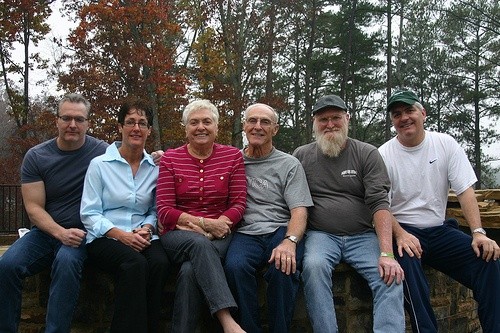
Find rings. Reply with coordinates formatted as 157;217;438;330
222;236;225;239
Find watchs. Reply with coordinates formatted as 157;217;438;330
281;236;301;246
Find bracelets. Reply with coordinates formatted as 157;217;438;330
473;228;486;235
379;251;394;259
143;225;153;241
204;232;215;241
199;216;208;231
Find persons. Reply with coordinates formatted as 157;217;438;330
224;102;315;333
375;90;500;333
80;103;170;333
0;93;163;333
292;95;408;333
158;99;248;333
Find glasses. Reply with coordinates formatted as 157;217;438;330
120;120;149;129
245;118;276;127
57;114;88;124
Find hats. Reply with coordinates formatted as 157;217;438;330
387;90;419;112
312;95;348;115
